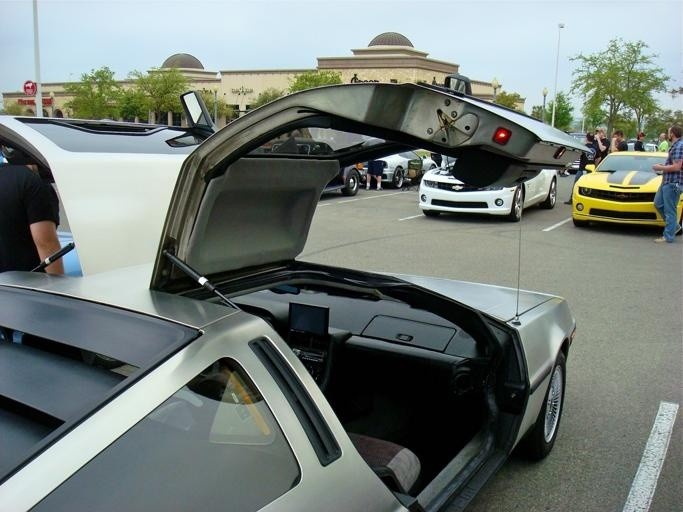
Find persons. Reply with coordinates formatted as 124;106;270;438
652;123;683;242
560;127;646;205
0;156;65;275
366;160;384;191
655;132;668;153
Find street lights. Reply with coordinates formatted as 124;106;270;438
551;22;567;127
490;78;499;104
541;86;549;123
212;83;218;127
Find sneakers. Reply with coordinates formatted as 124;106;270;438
674;224;682;236
653;235;667;244
563;199;572;205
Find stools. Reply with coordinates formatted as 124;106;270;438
344;427;426;498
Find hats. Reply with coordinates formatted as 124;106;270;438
637;132;646;137
585;132;595;142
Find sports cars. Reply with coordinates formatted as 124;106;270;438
0;74;595;512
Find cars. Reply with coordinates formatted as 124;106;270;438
416;159;560;222
624;142;659;152
270;137;361;197
357;151;438;189
571;150;683;235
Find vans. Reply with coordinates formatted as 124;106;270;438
566;132;588;172
